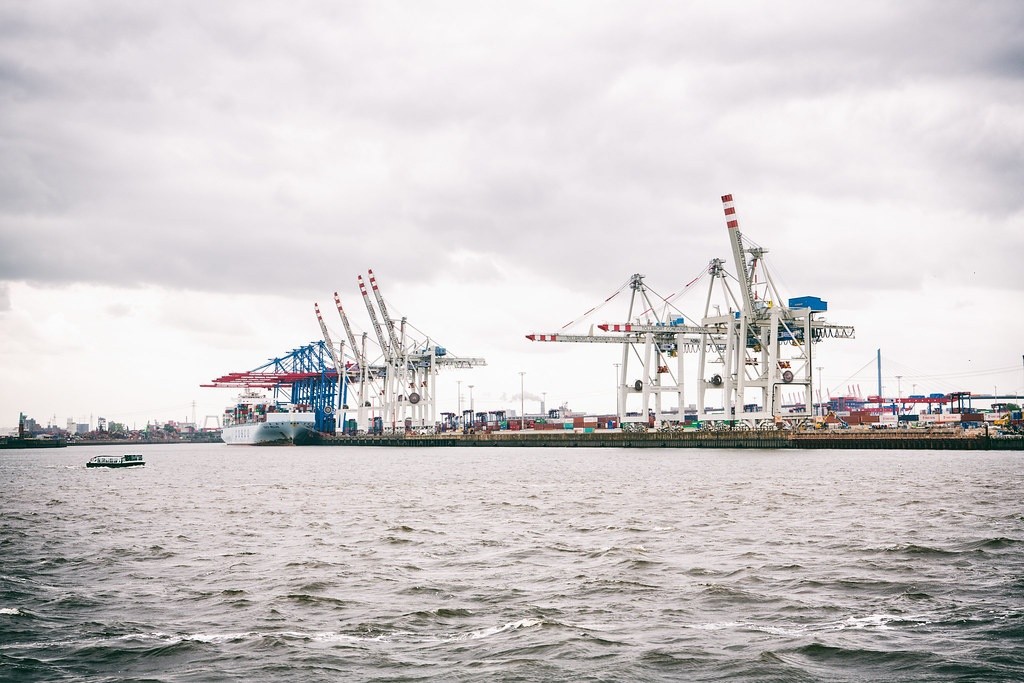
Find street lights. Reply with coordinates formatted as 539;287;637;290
456;379;462;418
896;376;903;398
613;362;621;413
518;369;527;419
816;366;825;403
468;384;476;412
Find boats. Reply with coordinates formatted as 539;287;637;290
0;423;68;448
220;392;317;446
85;453;147;470
